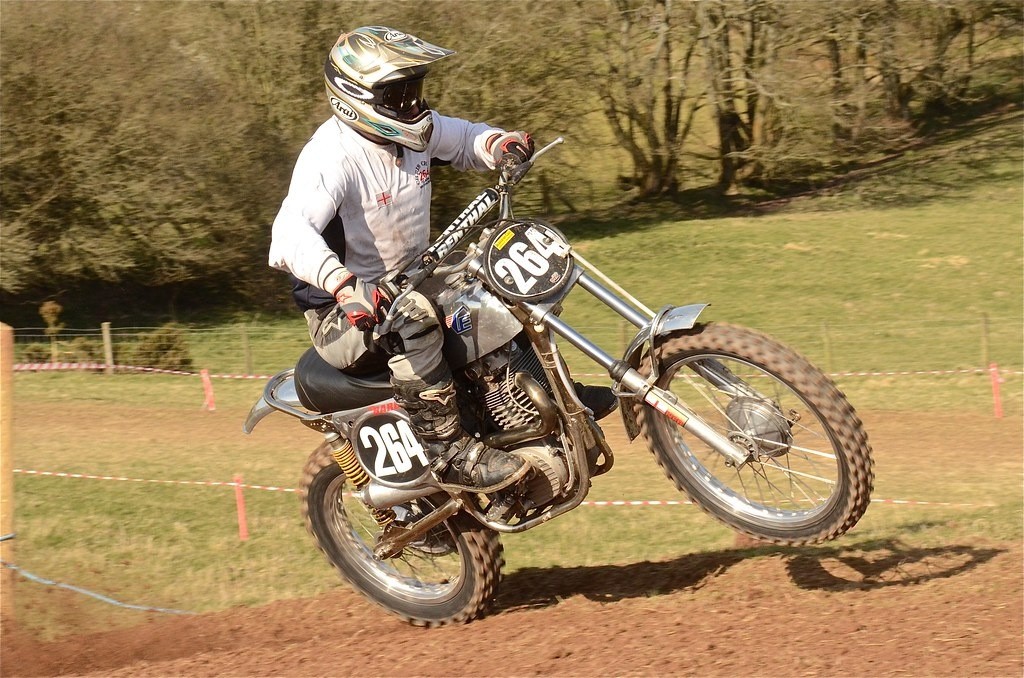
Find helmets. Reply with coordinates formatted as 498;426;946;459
323;24;456;153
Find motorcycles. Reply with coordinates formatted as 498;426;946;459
244;131;875;629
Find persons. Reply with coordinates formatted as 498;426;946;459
269;26;618;493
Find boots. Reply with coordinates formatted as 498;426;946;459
572;379;619;421
390;375;529;493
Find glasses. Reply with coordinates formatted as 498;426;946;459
383;77;421;113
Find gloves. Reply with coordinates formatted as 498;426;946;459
485;131;534;172
332;273;390;333
